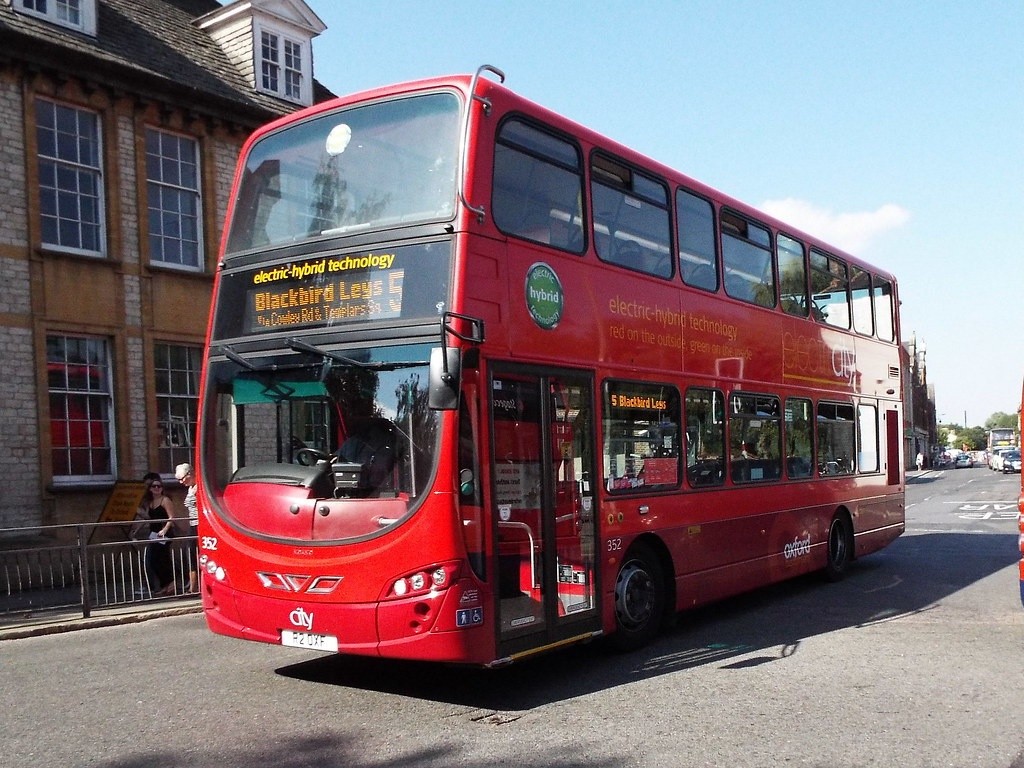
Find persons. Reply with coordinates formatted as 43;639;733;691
290;413;393;494
175;463;197;598
129;472;176;596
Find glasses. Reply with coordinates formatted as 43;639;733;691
178;473;188;481
151;484;162;489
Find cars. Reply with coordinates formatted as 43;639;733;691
1003;450;1022;474
988;446;1015;471
956;455;973;469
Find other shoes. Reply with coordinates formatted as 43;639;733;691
134;587;154;594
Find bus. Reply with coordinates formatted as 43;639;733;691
194;64;907;667
987;428;1016;469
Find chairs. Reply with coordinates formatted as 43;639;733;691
499;208;820;321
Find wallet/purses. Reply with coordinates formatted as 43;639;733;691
149;531;168;545
